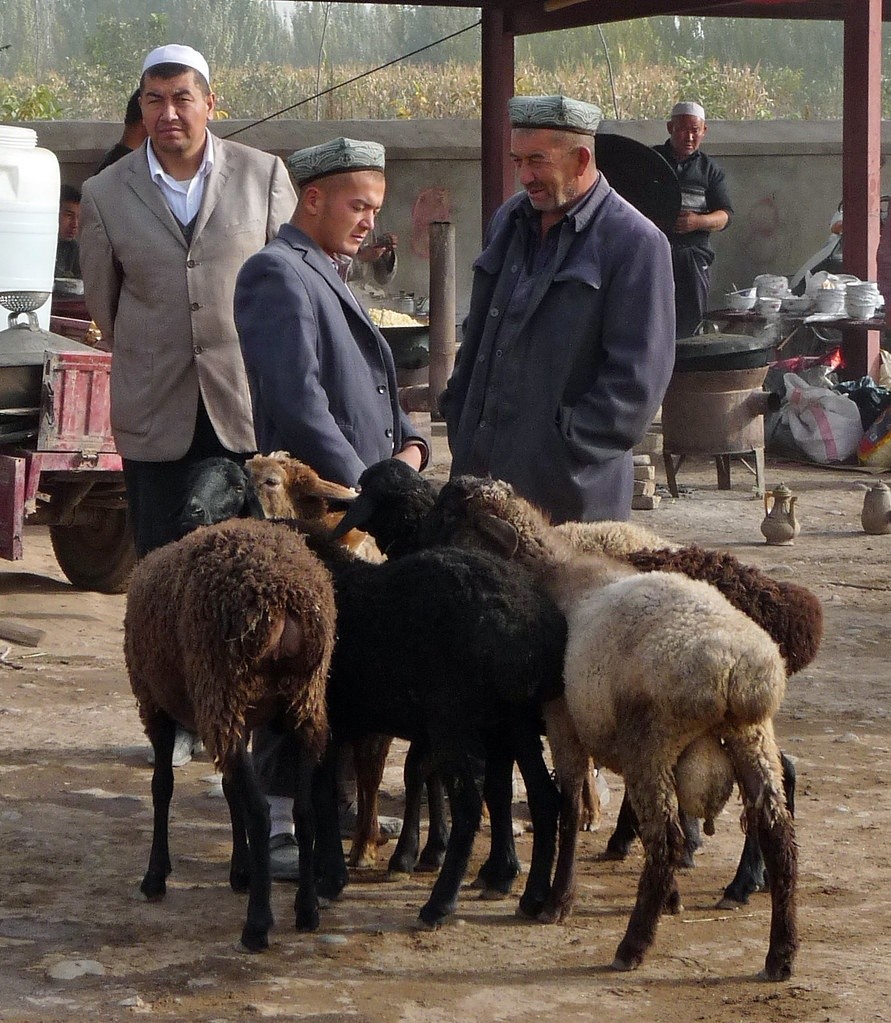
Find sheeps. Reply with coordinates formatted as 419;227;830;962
124;451;820;983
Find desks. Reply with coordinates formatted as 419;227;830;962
702;306;888;386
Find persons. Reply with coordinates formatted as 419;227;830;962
646;98;731;339
53;184;86;280
94;90;147;180
232;136;433;886
439;92;674;831
80;41;301;563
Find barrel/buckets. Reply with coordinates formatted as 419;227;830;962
0;122;63;337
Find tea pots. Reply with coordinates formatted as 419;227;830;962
760;482;800;546
862;479;891;535
387;290;428;317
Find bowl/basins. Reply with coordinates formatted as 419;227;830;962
723;293;758;312
752;271;879;321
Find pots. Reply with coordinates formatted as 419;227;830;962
672;319;777;373
359;292;387;315
590;130;683;240
1;310;107;410
374;322;432;370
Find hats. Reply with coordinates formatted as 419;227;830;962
141;44;209;85
671;102;705;119
286;135;386;188
508;94;601;136
